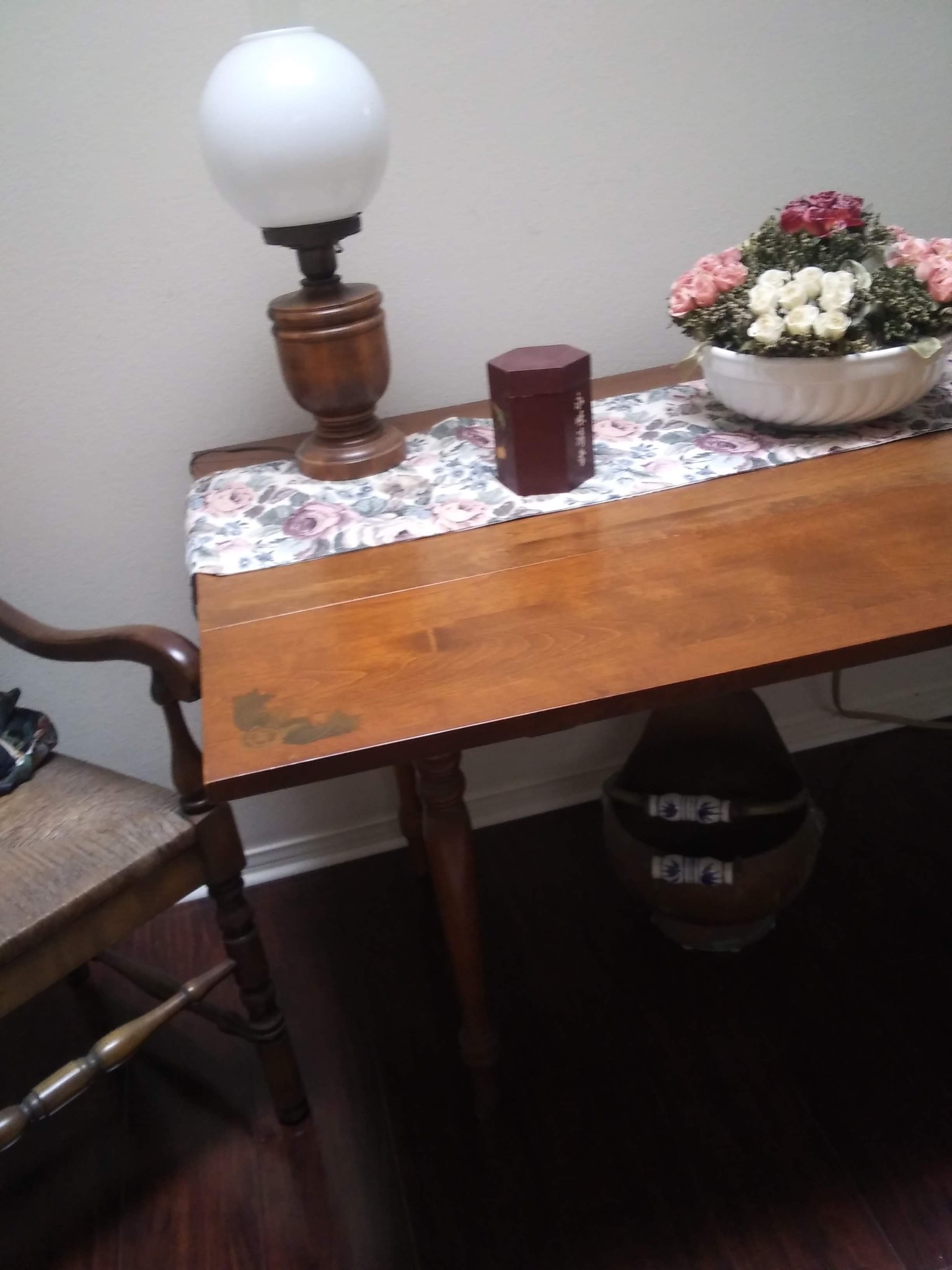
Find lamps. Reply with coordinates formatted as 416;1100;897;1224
202;26;407;478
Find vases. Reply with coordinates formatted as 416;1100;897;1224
699;332;948;427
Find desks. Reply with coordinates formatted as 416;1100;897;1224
187;359;952;1113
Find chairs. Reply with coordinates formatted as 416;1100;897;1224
0;601;307;1170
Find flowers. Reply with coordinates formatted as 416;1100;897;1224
671;187;952;357
